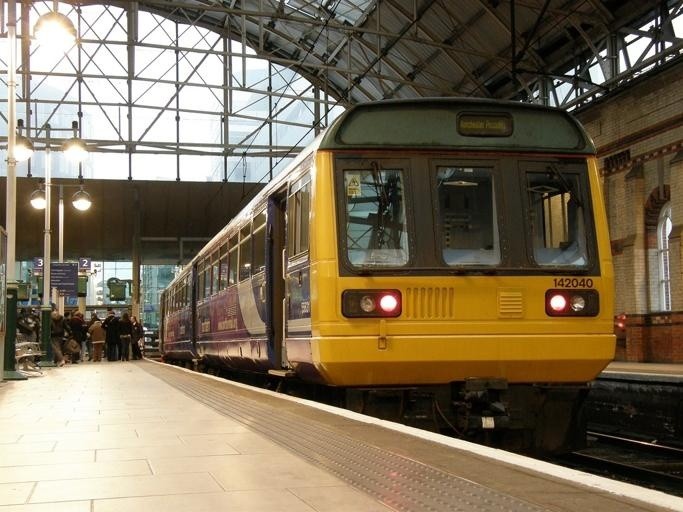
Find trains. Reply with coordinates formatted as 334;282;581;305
158;97;617;459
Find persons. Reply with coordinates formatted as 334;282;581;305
63;306;143;365
48;305;72;367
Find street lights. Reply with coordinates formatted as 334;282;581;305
3;0;77;380
30;177;92;263
16;118;89;367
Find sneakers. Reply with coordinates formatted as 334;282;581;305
53;354;143;368
